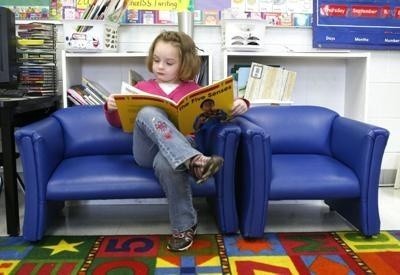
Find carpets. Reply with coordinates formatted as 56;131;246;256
0;229;400;275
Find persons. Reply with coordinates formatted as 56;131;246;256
103;28;252;252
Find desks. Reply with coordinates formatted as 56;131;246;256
0;94;68;236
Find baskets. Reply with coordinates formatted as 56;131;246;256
219;17;267;52
62;19;120;53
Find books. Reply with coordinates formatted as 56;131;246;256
112;75;235;136
66;0;130;48
67;69;145;107
230;60;298;104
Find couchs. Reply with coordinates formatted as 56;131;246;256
14;103;241;243
236;105;391;237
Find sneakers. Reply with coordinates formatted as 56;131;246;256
188;153;225;184
166;223;198;252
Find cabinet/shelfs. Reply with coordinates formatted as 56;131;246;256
222;48;372;123
61;49;213;110
